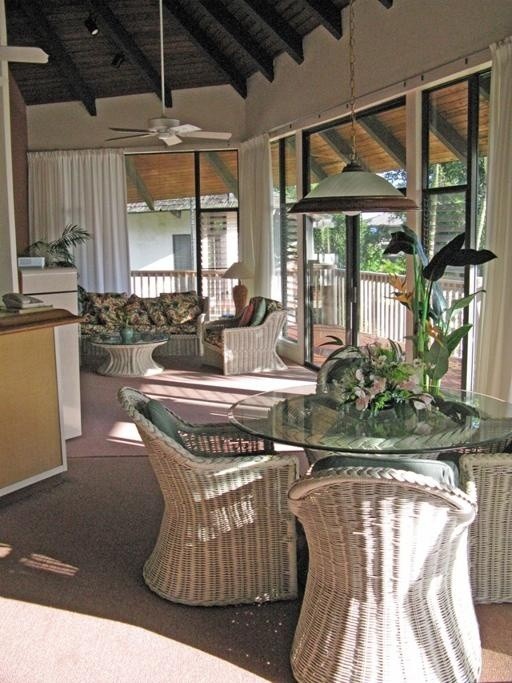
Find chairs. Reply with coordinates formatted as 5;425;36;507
202;298;288;374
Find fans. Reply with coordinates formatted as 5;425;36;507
106;2;231;145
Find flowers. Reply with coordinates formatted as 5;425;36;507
319;228;498;414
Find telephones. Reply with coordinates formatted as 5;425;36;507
2;293;53;309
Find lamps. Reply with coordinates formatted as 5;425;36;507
83;5;104;36
289;2;417;215
223;262;257;317
107;43;126;68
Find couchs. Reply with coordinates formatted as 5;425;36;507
78;293;211;370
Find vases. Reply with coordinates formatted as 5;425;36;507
119;317;134;342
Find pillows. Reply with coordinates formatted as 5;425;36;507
95;289;200;326
239;296;265;326
